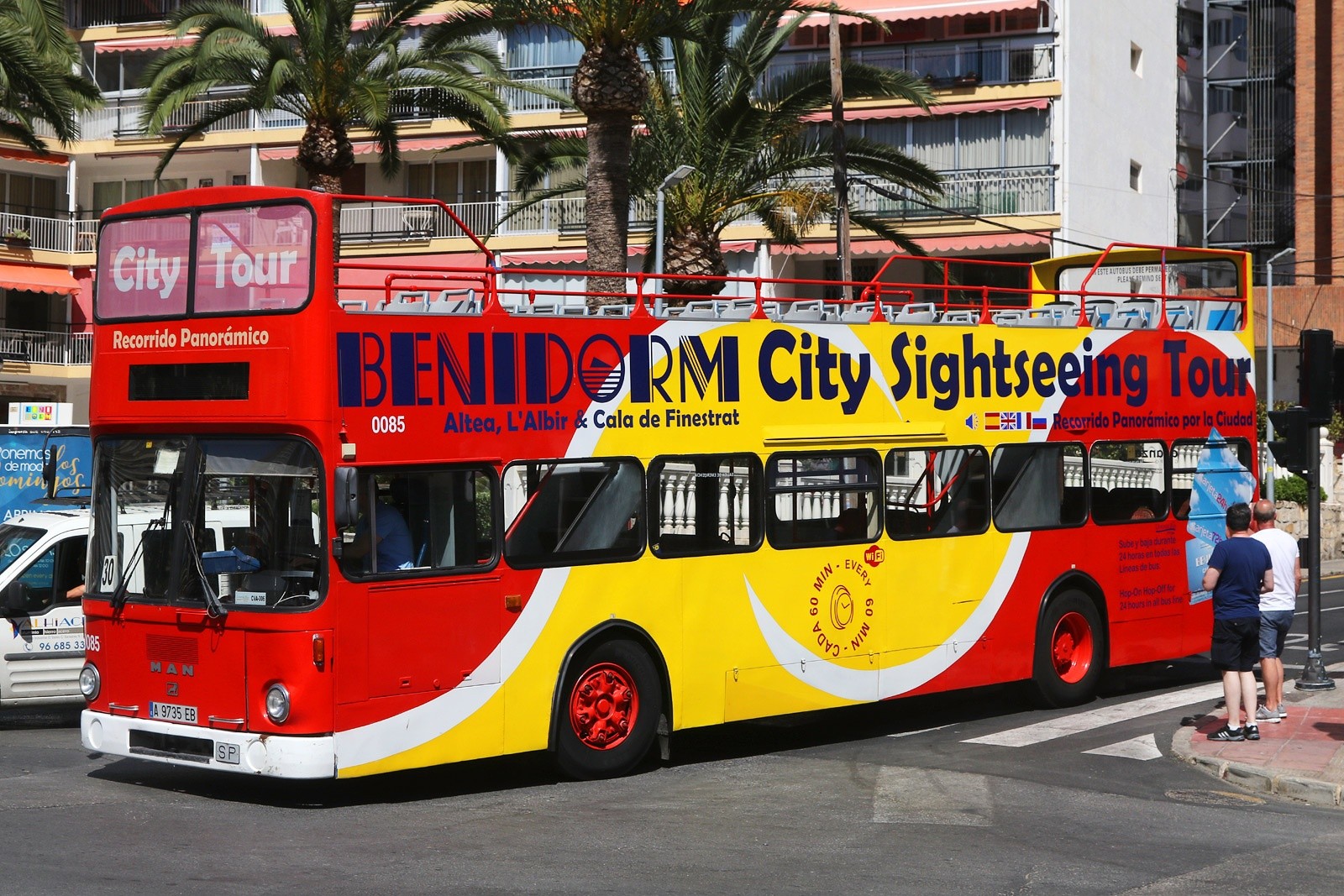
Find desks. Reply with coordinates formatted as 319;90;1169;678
77;231;97;253
0;332;45;360
401;210;440;242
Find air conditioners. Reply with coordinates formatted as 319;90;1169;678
1009;49;1050;79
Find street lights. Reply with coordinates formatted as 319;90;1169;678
654;166;696;318
1265;248;1296;503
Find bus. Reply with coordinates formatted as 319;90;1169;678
42;188;1260;777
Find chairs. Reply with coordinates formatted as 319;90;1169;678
1107;487;1161;521
257;279;1243;330
1061;485;1108;524
1161;489;1191;518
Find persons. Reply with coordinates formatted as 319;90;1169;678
1202;503;1274;741
339;478;415;576
1249;500;1302;723
818;509;865;540
54;547;85;603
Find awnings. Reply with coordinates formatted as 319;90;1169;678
0;262;82;295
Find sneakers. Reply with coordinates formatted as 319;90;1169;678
1245;704;1288;723
1207;725;1245;741
1243;725;1260;740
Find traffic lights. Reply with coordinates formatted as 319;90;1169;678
1267;407;1309;471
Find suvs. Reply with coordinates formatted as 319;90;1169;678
1;486;319;707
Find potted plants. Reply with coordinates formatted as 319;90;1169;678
0;227;32;248
